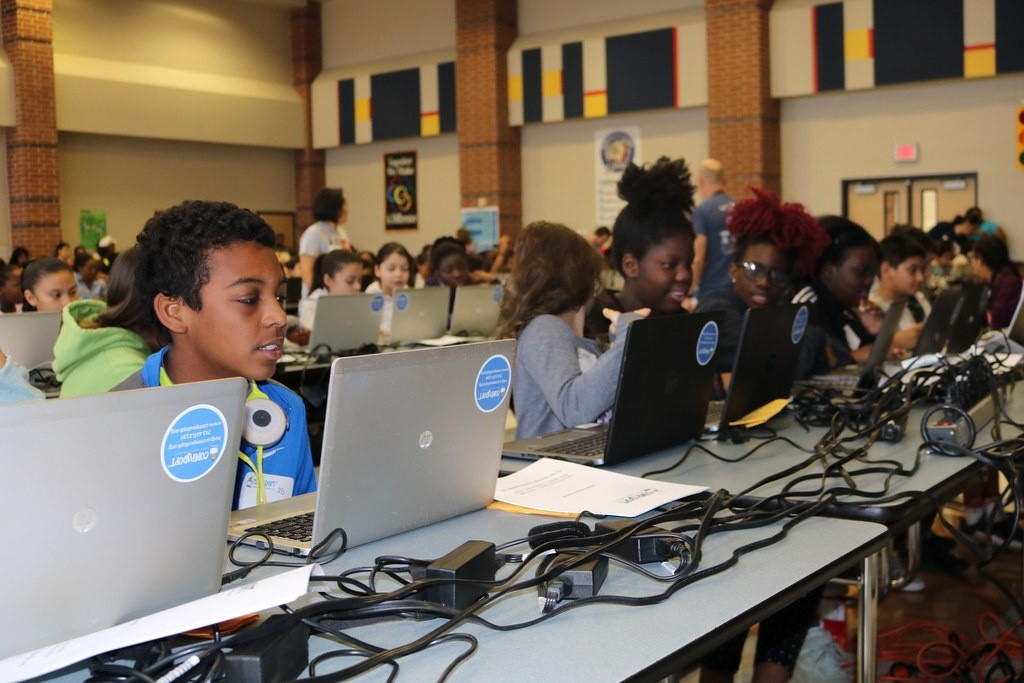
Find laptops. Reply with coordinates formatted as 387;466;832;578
1;270;1024;659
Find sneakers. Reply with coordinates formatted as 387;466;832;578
791;625;851;682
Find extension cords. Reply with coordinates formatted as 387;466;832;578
925;386;1002;448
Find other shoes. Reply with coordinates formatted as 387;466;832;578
895;531;966;572
958;504;1024;544
889;570;925;593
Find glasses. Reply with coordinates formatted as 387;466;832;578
734;260;791;286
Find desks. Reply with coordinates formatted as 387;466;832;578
0;248;1024;683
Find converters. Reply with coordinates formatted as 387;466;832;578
426;540;495;611
594;518;685;564
545;551;608;600
222;614;308;683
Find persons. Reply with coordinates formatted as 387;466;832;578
1;201;317;511
301;156;1024;683
